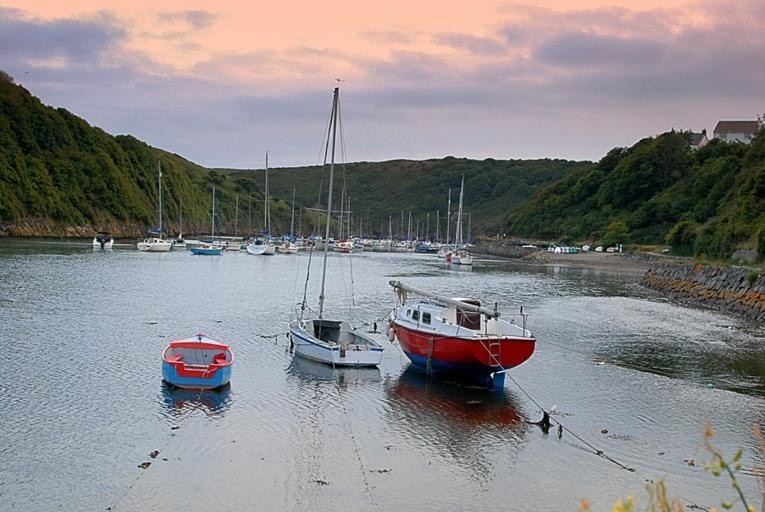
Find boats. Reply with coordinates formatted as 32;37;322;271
93;231;114;250
162;333;234;391
388;280;536;388
287;88;385;367
448;249;473;265
